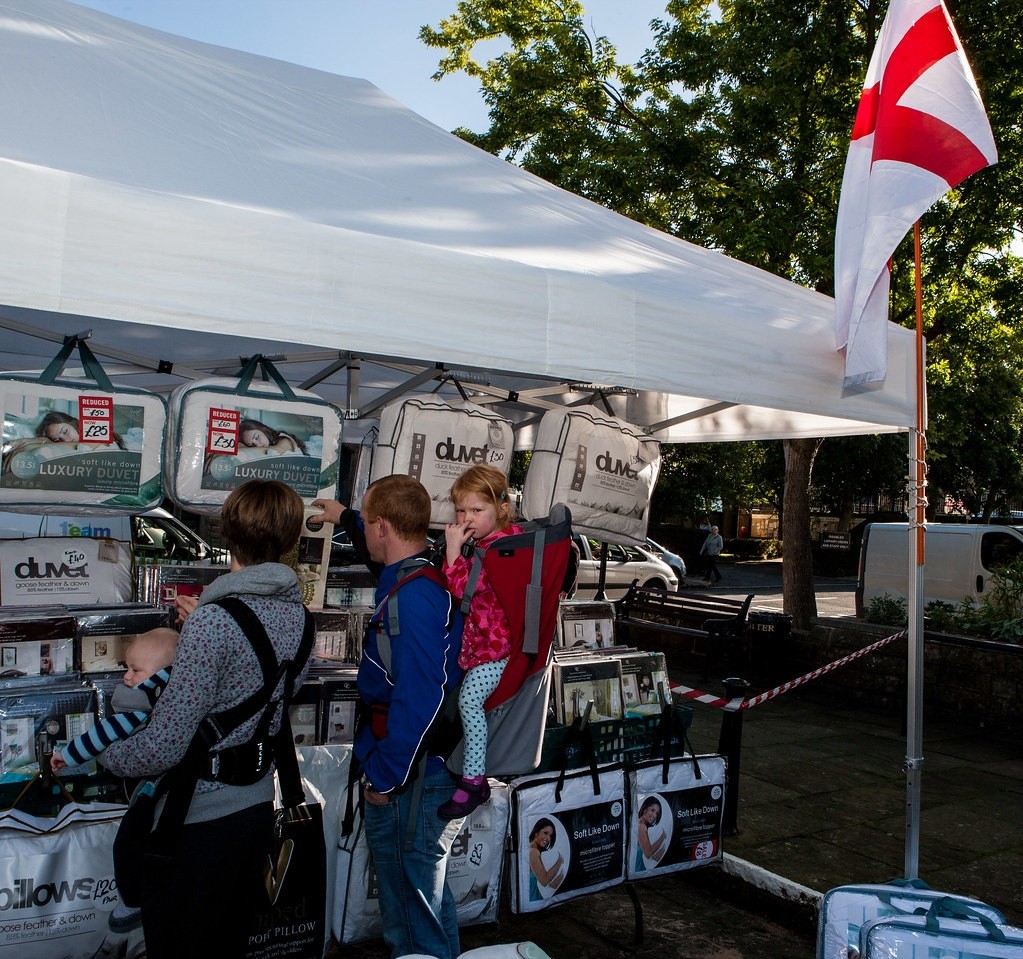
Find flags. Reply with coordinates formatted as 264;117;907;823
833;0;1000;397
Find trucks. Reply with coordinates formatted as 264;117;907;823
1;506;235;567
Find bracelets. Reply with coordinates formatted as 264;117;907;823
361;773;376;791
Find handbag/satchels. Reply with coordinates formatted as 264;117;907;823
815;877;1023;959
4;711;728;959
0;337;664;611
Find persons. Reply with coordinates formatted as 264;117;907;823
203;420;311;475
529;818;564;900
559;540;580;600
51;626;181;933
311;474;463;959
101;480;317;959
3;413;128;451
635;795;667;871
439;466;525;818
700;525;723;581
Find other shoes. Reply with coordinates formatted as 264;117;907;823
436;780;491;820
109;900;142;932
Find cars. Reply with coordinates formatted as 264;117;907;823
596;534;687;586
329;528;439;568
573;533;679;606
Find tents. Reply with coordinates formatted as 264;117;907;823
0;0;927;891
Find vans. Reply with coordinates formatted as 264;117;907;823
857;523;1022;622
